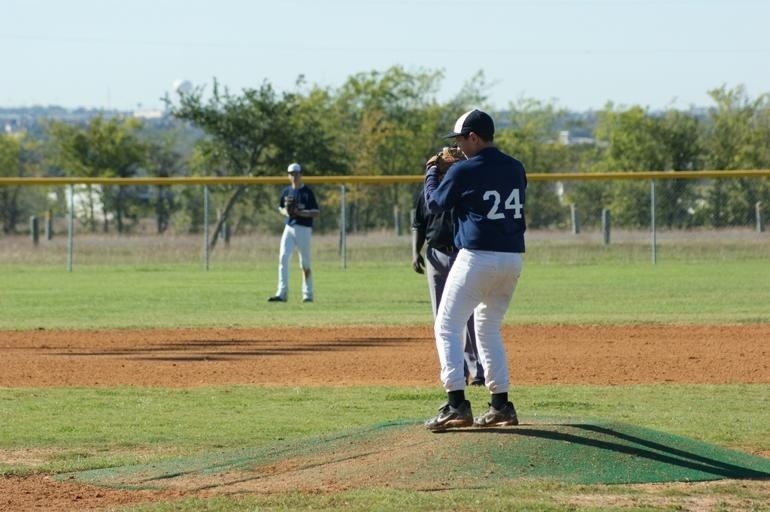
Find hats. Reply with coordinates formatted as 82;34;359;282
442;109;495;141
287;163;300;172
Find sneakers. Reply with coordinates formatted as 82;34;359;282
473;402;519;427
268;296;284;302
467;374;485;387
424;399;474;433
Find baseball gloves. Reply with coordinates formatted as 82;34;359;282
285;196;300;218
425;147;466;174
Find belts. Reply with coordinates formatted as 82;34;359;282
437;245;458;254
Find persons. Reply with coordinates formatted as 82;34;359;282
267;162;321;302
410;174;485;385
423;108;527;431
